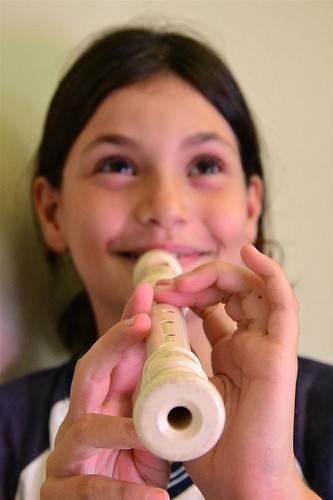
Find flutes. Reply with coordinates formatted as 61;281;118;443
132;248;229;460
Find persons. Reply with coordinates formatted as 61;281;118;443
2;26;333;500
